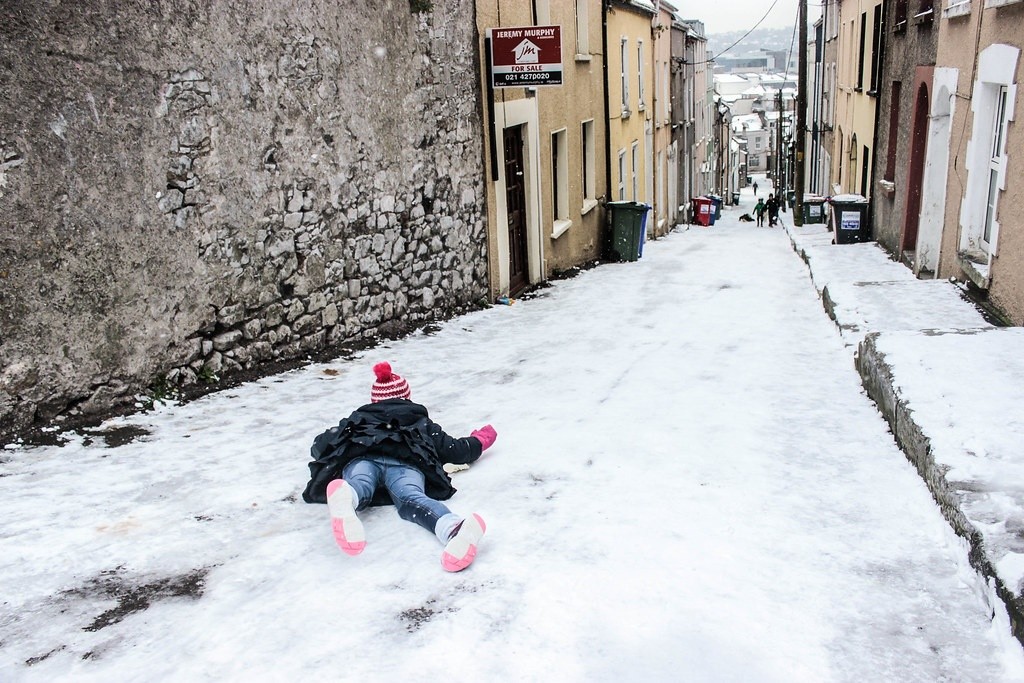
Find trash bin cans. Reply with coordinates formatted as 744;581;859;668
731;192;740;206
828;193;869;245
747;176;752;184
691;194;723;226
602;200;652;262
786;189;826;224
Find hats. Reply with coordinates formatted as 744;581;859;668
371;362;410;403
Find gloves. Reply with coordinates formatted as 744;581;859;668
470;424;497;451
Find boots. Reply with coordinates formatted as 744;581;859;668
435;513;486;573
327;479;367;556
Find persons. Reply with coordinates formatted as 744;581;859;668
739;213;755;222
302;361;497;573
752;198;767;227
761;194;780;228
752;181;759;196
